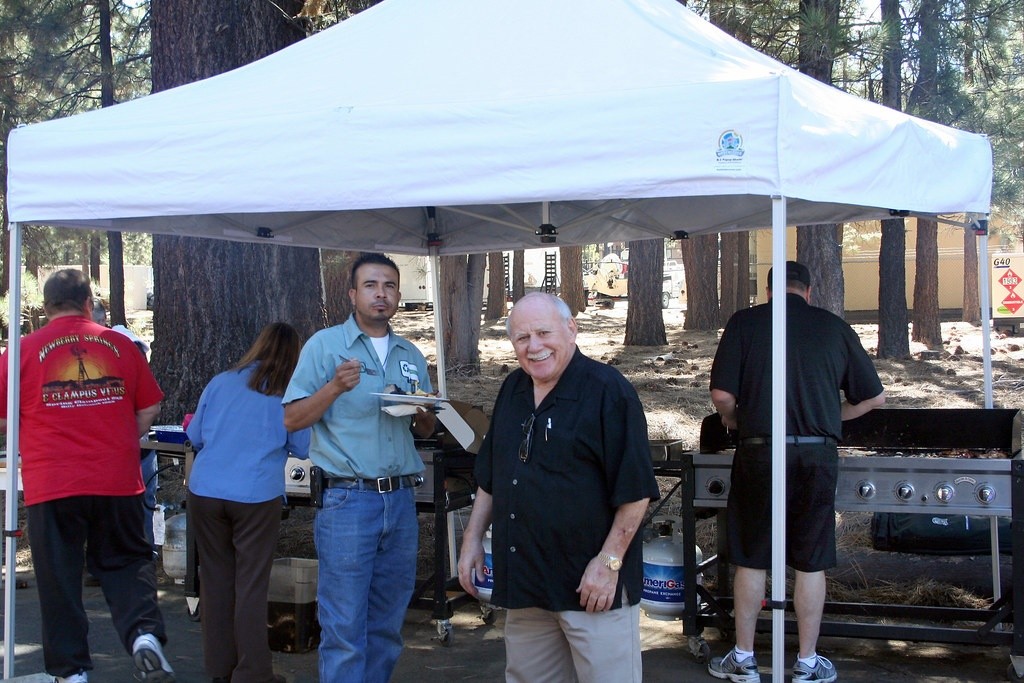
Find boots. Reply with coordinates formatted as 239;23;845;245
154;545;175;589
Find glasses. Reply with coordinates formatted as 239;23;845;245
517;413;535;463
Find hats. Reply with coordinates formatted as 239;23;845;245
767;260;810;287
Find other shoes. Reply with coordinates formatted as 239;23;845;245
84;575;101;586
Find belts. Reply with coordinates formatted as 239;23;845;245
324;474;414;494
738;435;835;446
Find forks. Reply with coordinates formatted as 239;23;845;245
338;355;378;377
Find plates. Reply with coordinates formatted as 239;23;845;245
370;390;450;406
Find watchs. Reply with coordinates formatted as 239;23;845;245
598;553;622;571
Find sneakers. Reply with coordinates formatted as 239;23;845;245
54;669;88;683
791;655;838;683
707;647;761;683
132;633;178;683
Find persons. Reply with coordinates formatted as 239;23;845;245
83;297;174;589
708;261;885;682
281;253;436;683
186;323;311;680
457;293;659;683
0;269;176;683
